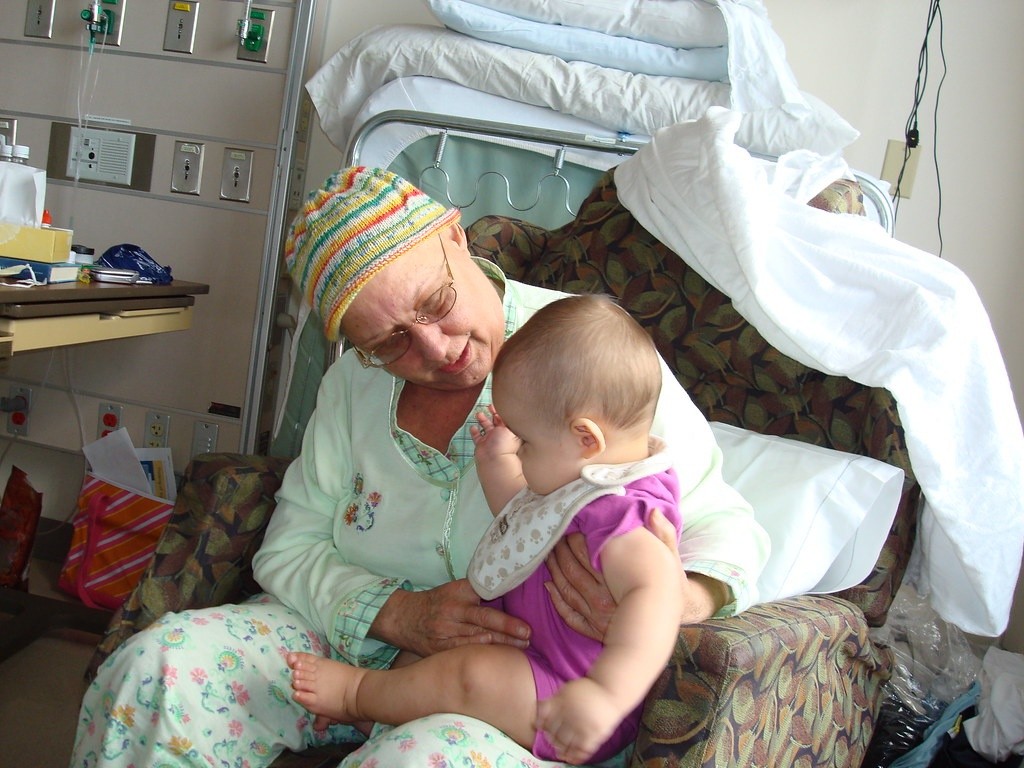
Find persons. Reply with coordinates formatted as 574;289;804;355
68;164;771;768
285;291;684;767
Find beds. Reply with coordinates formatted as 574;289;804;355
266;75;893;480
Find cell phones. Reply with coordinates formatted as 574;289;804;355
89;268;153;285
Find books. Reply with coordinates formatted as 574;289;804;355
0;256;81;284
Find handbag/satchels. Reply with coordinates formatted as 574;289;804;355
58;468;177;613
0;465;43;593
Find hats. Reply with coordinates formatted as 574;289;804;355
285;167;462;342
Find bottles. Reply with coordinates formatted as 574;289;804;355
11;145;30;165
0;145;13;162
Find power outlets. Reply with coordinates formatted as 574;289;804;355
219;147;256;203
24;0;56;40
90;0;127;46
143;411;171;448
236;0;276;63
5;385;33;438
96;402;124;439
169;140;206;196
162;0;201;53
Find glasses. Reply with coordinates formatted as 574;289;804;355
351;232;458;369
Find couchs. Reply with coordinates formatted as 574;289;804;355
82;120;990;768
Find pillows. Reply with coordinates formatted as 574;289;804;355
467;0;728;49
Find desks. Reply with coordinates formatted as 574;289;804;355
0;278;212;357
